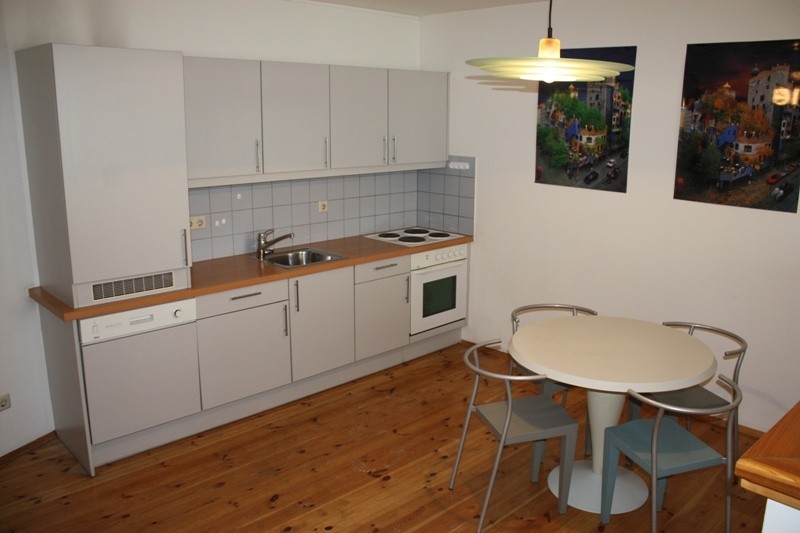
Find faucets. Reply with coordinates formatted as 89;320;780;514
257;229;294;262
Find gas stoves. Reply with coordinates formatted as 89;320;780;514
363;227;465;248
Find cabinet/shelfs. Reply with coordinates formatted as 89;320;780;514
12;42;477;477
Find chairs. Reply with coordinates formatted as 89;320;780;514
449;303;748;533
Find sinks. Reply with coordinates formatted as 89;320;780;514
249;247;351;269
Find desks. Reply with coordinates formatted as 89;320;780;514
507;317;716;515
734;399;800;533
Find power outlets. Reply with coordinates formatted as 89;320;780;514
190;215;204;229
319;200;328;213
0;393;11;412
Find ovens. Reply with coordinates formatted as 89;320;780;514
410;243;468;335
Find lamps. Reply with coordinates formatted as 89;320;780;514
465;0;635;83
